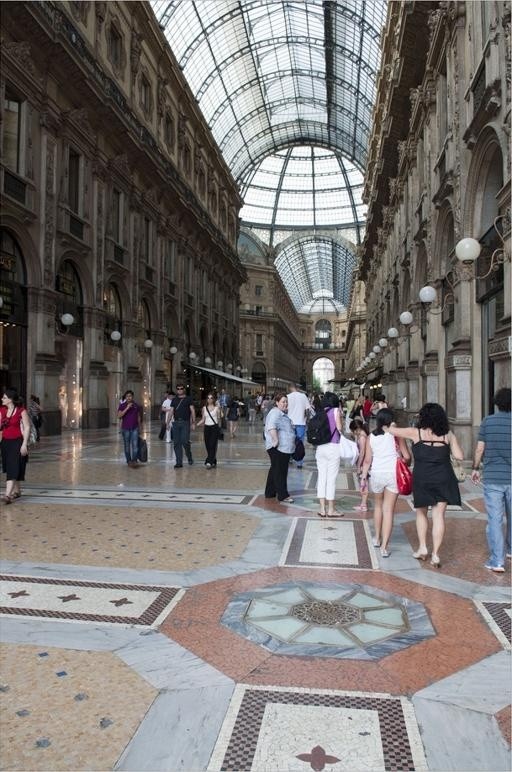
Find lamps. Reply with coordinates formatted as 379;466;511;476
143;329;154;348
400;311;422;334
187;346;197;359
455;238;512;283
103;323;122;341
217;353;223;367
55;313;74;326
204;350;212;364
237;360;248;374
388;328;413;347
227;359;233;369
169;340;178;354
418;286;454;316
356;339;388;376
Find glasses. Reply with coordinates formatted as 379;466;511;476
177;388;183;390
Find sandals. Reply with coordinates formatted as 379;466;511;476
1;492;21;501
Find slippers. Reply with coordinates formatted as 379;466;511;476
318;509;344;517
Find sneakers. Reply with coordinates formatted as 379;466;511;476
173;459;216;468
285;498;296;503
349;412;355;418
373;536;512;571
128;462;138;468
297;465;303;468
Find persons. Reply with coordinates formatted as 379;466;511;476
370;395;378;419
271;394;274;406
117;390;141;464
320;393;324;400
265;393;297;504
381;402;464;570
257;392;260;407
118;394;126;434
261;396;270;425
363;396;373;435
338;394;343;412
286;382;309;469
245;392;252;421
312;395;321;439
166;384;197;468
158;391;173;440
248;395;257;425
353;399;365;428
212;385;220;407
226;396;240;438
258;394;263;410
349;419;373;511
161;393;175;443
471;387;511;571
372;395;387;429
195;391;224;470
307;390;315;425
219;388;230;429
29;394;43;441
362;407;411;557
241;399;246;417
316;391;345;516
346;394;355;440
0;390;31;503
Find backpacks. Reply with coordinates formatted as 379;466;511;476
307;406;339;446
370;401;379;415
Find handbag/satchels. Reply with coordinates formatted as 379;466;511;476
292;438;305;461
451;460;465;482
138;436;147;462
396;457;412;495
218;417;227;428
32;416;42;428
305;407;316;419
218;434;224;440
340;435;359;459
20;418;37;445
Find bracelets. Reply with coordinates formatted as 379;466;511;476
472;464;479;470
192;422;195;425
